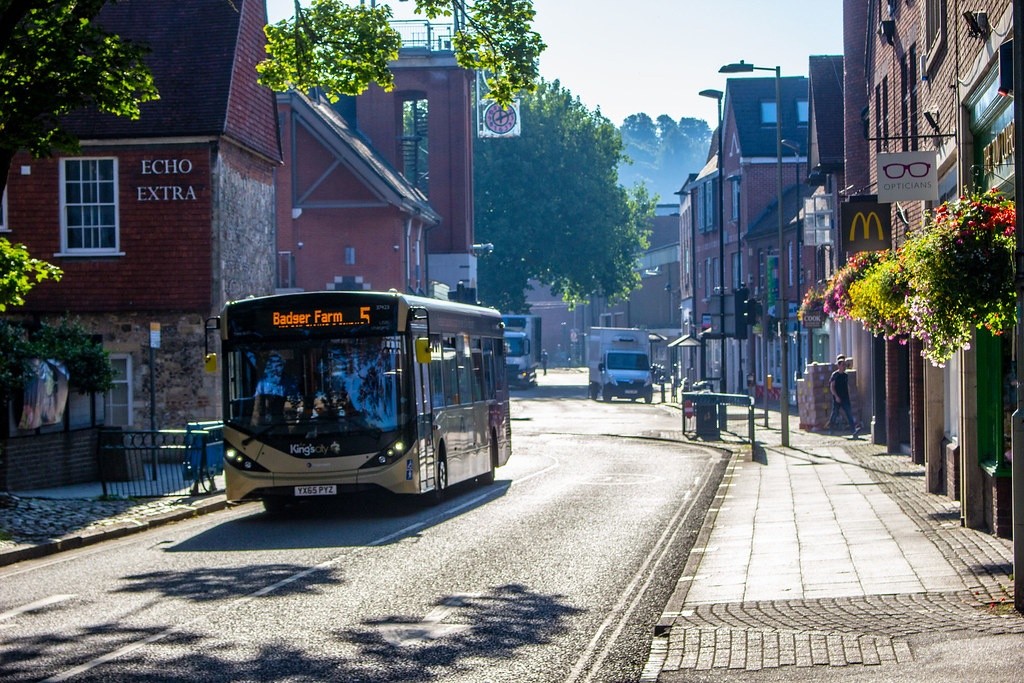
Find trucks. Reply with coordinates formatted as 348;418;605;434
588;327;656;404
500;315;542;389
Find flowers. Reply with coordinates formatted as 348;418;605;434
794;184;1021;369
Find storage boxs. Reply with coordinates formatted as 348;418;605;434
796;357;861;433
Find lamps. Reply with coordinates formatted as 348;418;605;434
344;244;356;266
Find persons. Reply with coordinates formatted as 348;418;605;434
822;354;862;436
541;349;548;375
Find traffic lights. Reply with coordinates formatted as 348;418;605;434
743;300;755;325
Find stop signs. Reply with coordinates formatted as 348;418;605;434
683;401;693;418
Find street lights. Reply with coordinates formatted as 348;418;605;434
699;89;727;429
781;141;802;407
674;189;694;387
561;322;566;352
718;61;790;447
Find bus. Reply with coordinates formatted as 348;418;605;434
204;286;512;517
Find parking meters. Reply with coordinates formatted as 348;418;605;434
650;363;665;384
684;380;689;394
746;373;755;396
660;376;665;403
